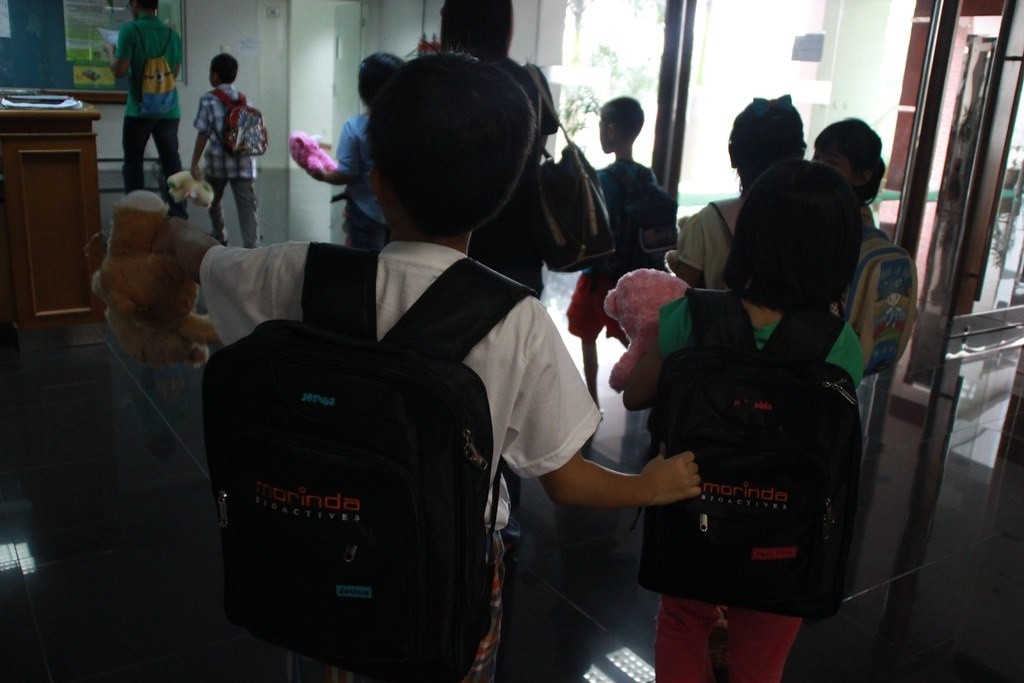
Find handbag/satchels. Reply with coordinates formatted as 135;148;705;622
526;63;615;273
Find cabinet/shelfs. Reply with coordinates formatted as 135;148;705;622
0;88;107;330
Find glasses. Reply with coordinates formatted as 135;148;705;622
125;2;131;10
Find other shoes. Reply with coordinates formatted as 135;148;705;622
223;240;228;246
499;516;521;551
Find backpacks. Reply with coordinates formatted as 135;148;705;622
629;289;862;624
129;22;178;115
203;243;540;682
837;226;918;378
603;166;678;286
207;89;268;158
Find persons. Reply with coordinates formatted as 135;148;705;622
103;0;190;222
157;0;916;683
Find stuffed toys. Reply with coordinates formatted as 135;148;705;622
604;268;692;393
290;137;337;175
167;170;214;208
93;190;221;369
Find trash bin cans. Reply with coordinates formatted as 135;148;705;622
0;93;104;334
